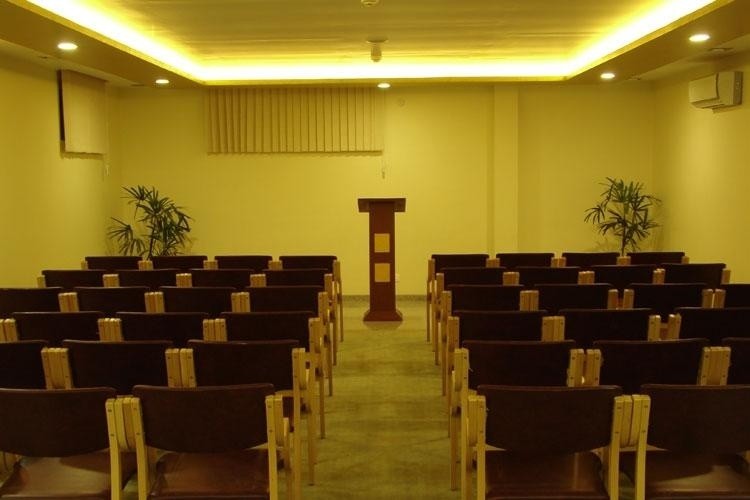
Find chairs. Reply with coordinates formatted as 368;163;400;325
425;248;749;499
0;253;345;500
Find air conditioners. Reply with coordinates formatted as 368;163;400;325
689;68;743;110
